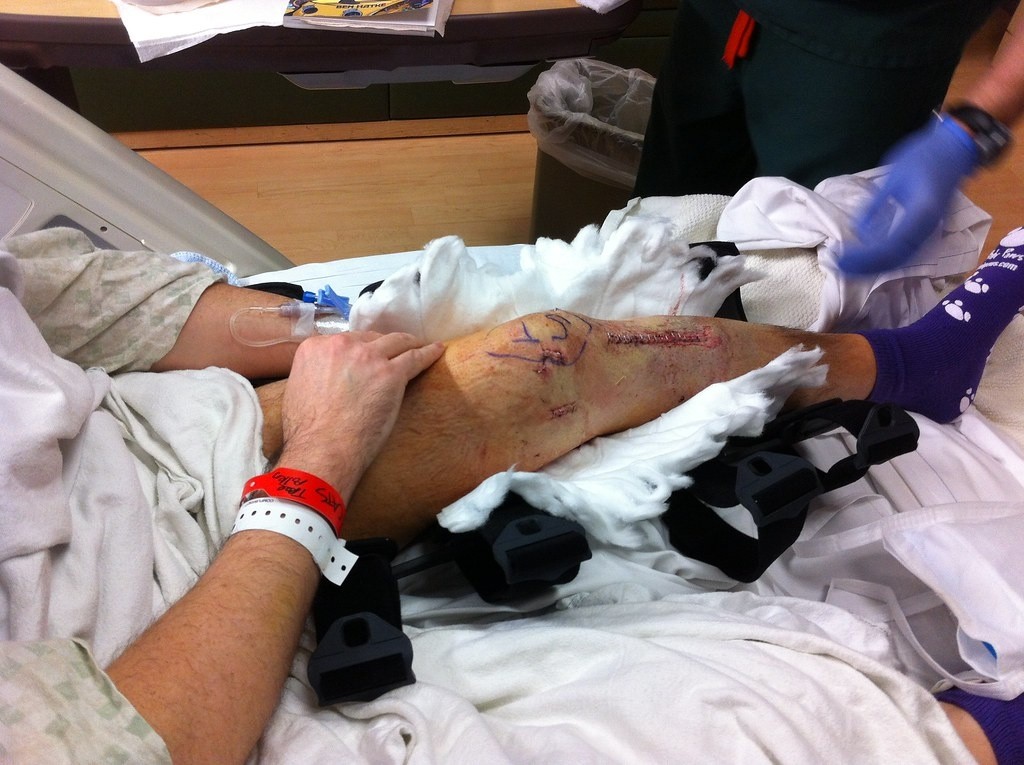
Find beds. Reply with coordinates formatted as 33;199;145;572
0;64;1024;694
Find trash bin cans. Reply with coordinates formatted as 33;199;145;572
529;54;657;244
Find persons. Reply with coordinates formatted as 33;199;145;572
632;0;1024;272
0;210;1024;765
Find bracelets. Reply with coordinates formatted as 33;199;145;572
239;468;345;536
230;497;358;585
950;104;1010;162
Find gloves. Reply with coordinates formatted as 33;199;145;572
836;111;980;275
